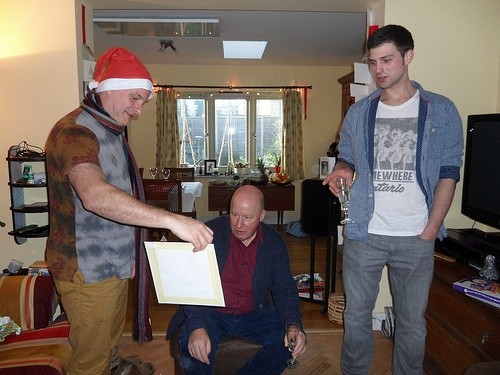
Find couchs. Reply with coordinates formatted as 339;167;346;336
0;276;73;375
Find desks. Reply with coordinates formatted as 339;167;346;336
181;181;203;220
208;180;295;234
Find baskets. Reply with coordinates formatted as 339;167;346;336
328;293;346;325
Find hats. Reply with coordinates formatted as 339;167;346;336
88;48;154;100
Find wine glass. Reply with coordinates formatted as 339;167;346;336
286;322;300;369
149;167;158;186
334;177;356;224
162;169;170;189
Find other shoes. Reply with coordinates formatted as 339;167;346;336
110;357;154;375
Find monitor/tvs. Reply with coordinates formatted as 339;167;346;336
461;114;500;230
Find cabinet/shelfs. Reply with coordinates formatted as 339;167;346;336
7;144;50;244
425;228;500;375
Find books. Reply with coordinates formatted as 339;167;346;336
454;276;500;304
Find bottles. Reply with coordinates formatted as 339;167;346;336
28;168;34;184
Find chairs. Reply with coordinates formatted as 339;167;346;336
141;178;182;240
164;167;194;182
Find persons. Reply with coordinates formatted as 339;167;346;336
165;184;307;375
45;48;214;375
322;24;464;375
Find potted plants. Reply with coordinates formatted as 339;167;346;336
268;151;281;173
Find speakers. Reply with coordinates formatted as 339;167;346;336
300;178;341;236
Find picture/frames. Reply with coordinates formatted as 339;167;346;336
205;159;216;175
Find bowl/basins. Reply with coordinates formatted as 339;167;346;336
233;168;250;175
270;180;294;186
208;179;226;185
226;179;240;186
